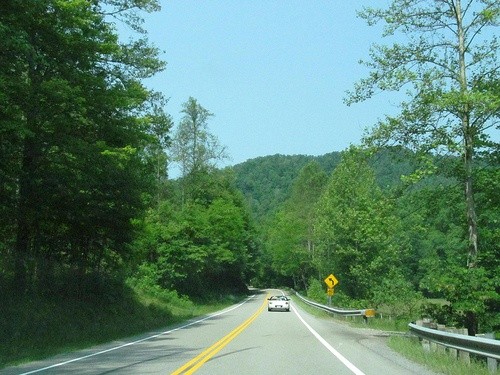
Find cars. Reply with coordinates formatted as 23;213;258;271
267;296;291;312
